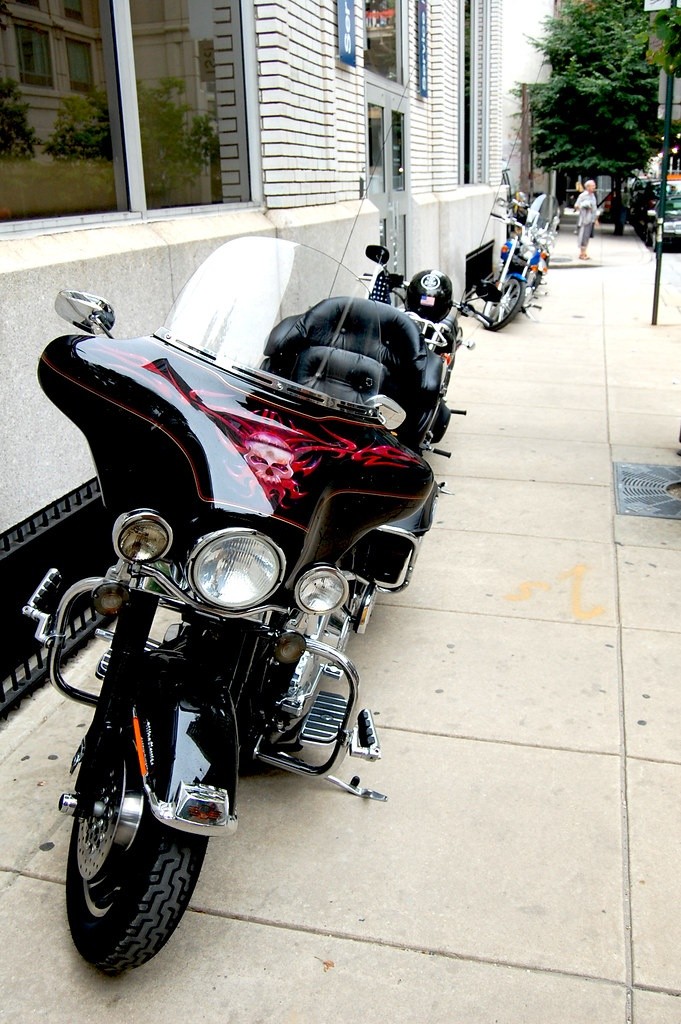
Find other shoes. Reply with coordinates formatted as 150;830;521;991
580;255;591;261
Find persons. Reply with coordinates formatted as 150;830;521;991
610;191;625;235
624;182;679;224
573;180;597;261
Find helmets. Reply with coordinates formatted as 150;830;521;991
407;270;454;322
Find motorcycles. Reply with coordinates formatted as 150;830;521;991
353;244;503;416
19;233;451;979
472;192;562;331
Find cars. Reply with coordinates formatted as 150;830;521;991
592;169;681;251
